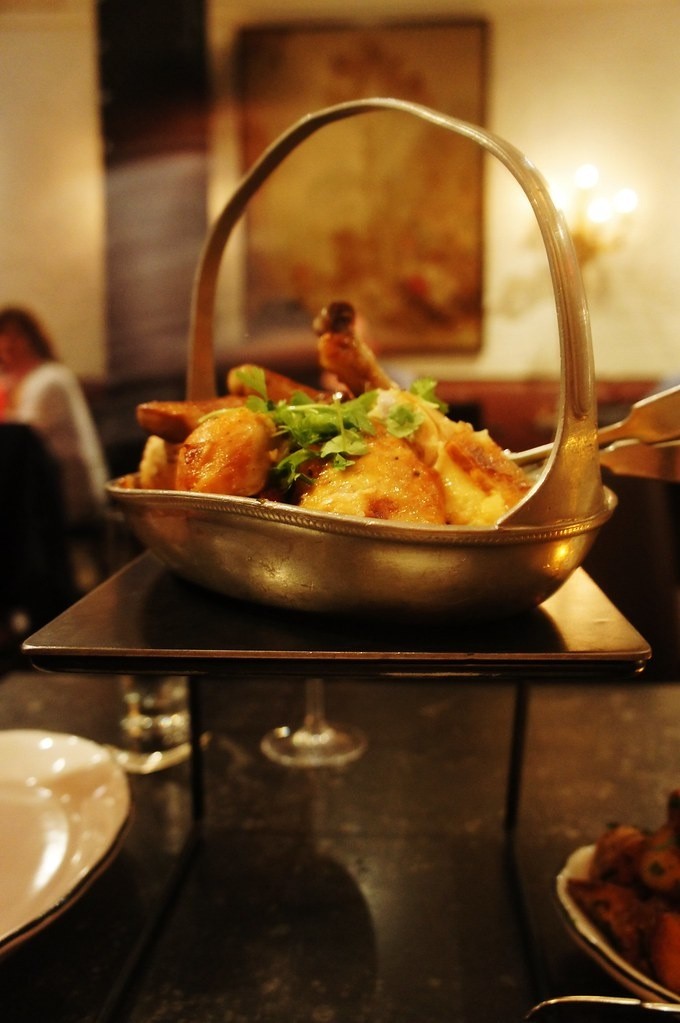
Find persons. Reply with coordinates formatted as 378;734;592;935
0;421;89;664
0;305;113;587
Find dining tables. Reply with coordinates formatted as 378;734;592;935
0;670;680;1022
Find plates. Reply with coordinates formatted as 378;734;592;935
1;722;134;963
555;830;680;1008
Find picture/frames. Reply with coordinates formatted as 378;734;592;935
239;17;486;357
